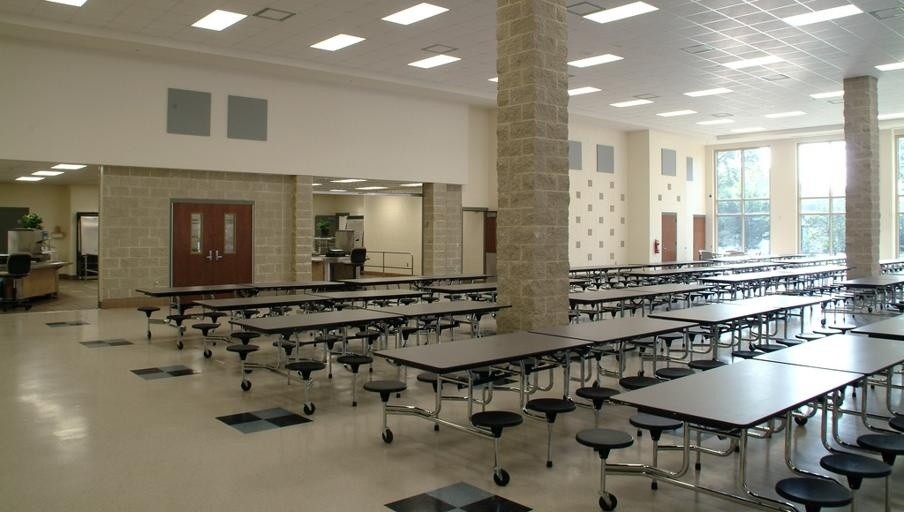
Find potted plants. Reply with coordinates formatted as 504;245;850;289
316;220;330;239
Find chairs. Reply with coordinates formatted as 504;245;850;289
0;253;33;314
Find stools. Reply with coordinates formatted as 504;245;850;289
125;249;904;422
469;409;523;487
523;396;575;471
360;380;409;444
776;477;853;512
856;431;904;471
817;452;892;492
574;426;634;511
628;411;683;493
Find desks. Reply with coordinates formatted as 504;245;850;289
371;329;594;486
0;259;74;311
603;355;866;512
